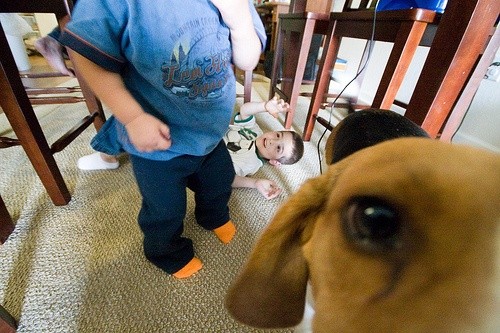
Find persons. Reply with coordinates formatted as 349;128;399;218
62;0;267;278
32;12;75;77
77;96;304;198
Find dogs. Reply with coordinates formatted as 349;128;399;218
225;136;500;333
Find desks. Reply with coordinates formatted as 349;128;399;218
303;8;442;149
269;11;363;129
